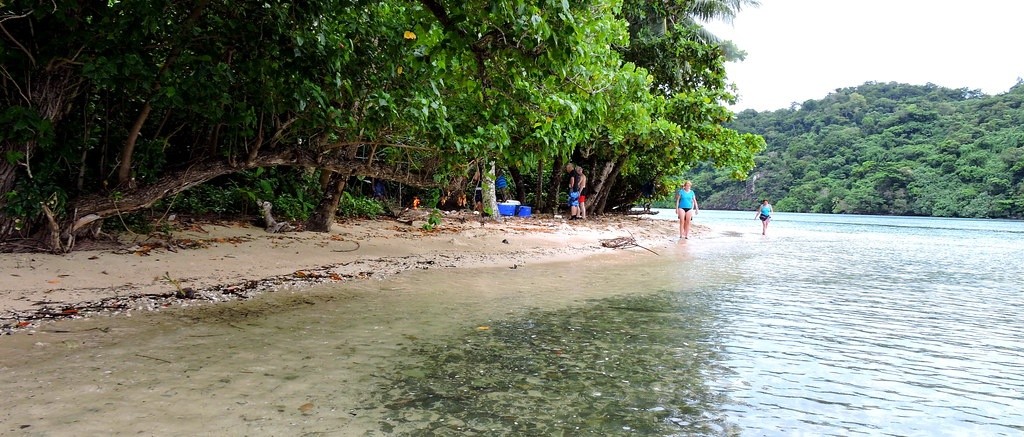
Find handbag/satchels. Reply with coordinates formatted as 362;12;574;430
760;216;767;221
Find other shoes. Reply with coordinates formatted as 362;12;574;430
569;215;576;220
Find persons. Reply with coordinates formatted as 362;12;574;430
574;165;587;220
676;181;700;240
754;199;774;235
494;169;508;202
566;163;580;221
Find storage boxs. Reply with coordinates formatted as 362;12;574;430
498;199;532;216
